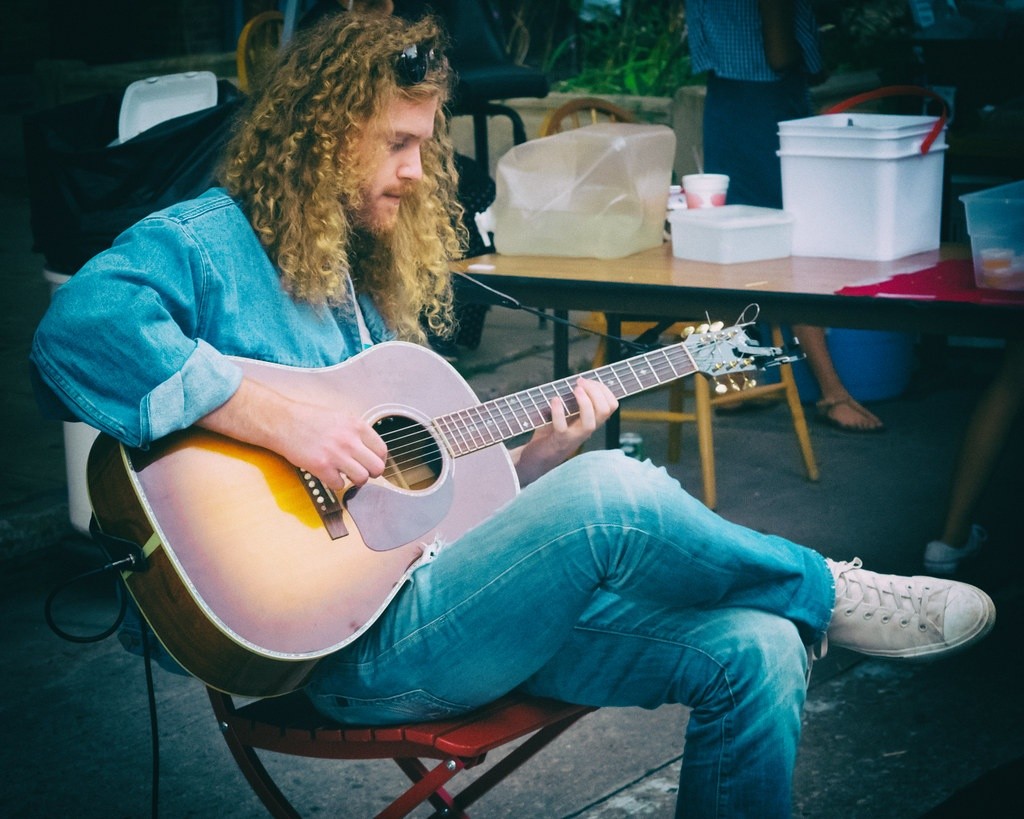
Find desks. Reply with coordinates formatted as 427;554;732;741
450;244;1023;448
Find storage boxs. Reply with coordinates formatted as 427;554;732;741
959;180;1024;292
776;86;948;261
667;204;792;263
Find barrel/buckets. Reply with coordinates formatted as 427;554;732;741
44;268;102;538
776;85;948;262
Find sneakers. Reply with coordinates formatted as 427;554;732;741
809;557;996;661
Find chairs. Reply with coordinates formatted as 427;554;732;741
442;99;527;257
541;97;819;510
237;11;285;94
205;681;604;819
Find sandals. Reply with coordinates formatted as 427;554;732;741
816;397;886;433
713;393;780;417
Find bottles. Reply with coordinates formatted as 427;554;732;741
664;186;687;240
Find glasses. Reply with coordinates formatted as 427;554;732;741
367;39;443;85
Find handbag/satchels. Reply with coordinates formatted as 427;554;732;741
758;0;832;86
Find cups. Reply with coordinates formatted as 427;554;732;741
683;174;730;209
981;248;1012;286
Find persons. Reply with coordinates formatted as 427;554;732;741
28;9;997;819
686;3;883;431
918;322;1023;568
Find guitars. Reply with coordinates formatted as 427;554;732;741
85;299;810;698
